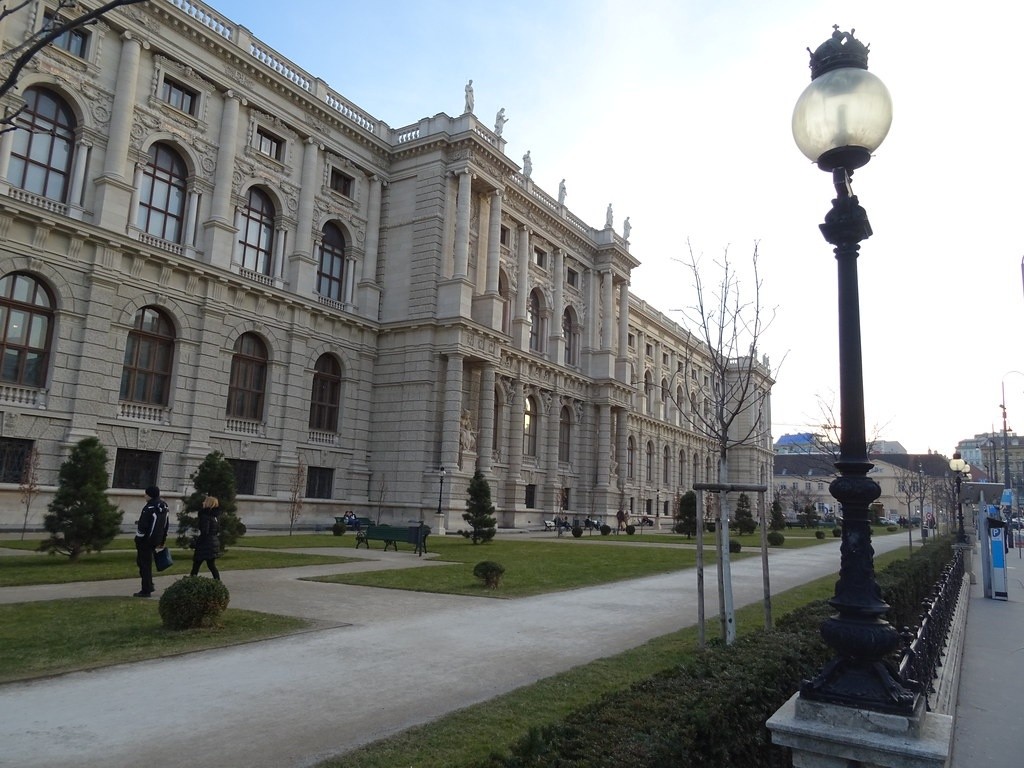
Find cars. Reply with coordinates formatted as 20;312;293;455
836;515;922;526
1004;517;1024;530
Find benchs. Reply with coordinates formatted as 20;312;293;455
637;518;648;526
583;520;600;530
543;520;563;531
787;522;806;529
332;517;376;536
356;525;431;554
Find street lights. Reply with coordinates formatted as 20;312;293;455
434;466;447;515
791;23;916;718
949;451;967;544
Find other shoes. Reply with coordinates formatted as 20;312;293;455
150;586;154;592
133;591;150;598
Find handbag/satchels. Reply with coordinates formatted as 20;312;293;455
190;535;197;549
343;511;357;525
153;547;173;572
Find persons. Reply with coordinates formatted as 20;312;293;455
344;511;361;531
131;486;169;597
617;510;653;532
190;497;222;581
559;179;567;204
606;203;613;228
522;150;533;176
493;108;509;137
899;516;906;529
464;80;475;113
554;515;603;531
623;217;632;241
609;444;619;476
459;409;479;451
750;343;770;368
